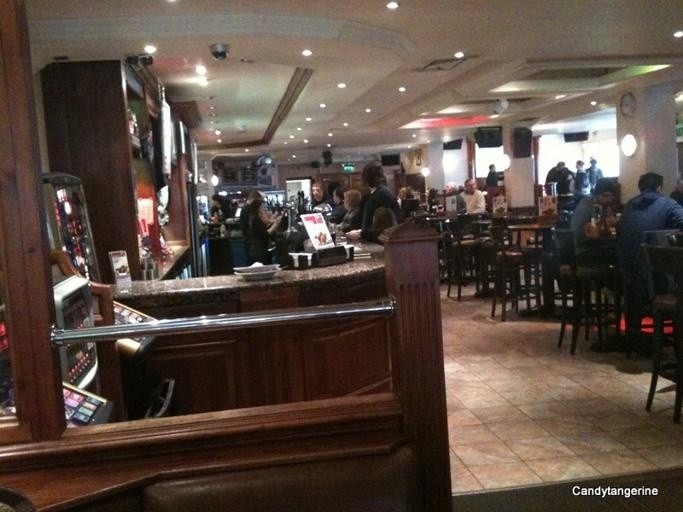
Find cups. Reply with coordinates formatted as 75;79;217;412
141;256;164;281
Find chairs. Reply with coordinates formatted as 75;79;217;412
415;213;683;427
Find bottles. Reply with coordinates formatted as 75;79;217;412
326;222;336;244
127;106;136;137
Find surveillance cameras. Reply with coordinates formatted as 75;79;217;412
209;43;229;61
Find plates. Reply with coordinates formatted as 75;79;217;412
232;263;281;280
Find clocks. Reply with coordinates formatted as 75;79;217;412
620;93;636;116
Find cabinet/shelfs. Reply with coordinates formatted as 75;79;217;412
40;59;205;282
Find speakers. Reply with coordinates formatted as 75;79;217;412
474;130;502;148
381;154;400;166
511;127;532;158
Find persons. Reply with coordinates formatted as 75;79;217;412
486;164;503;187
208;191;287;266
459;179;485;216
444;182;466;216
545;157;682;339
309;166;420;245
